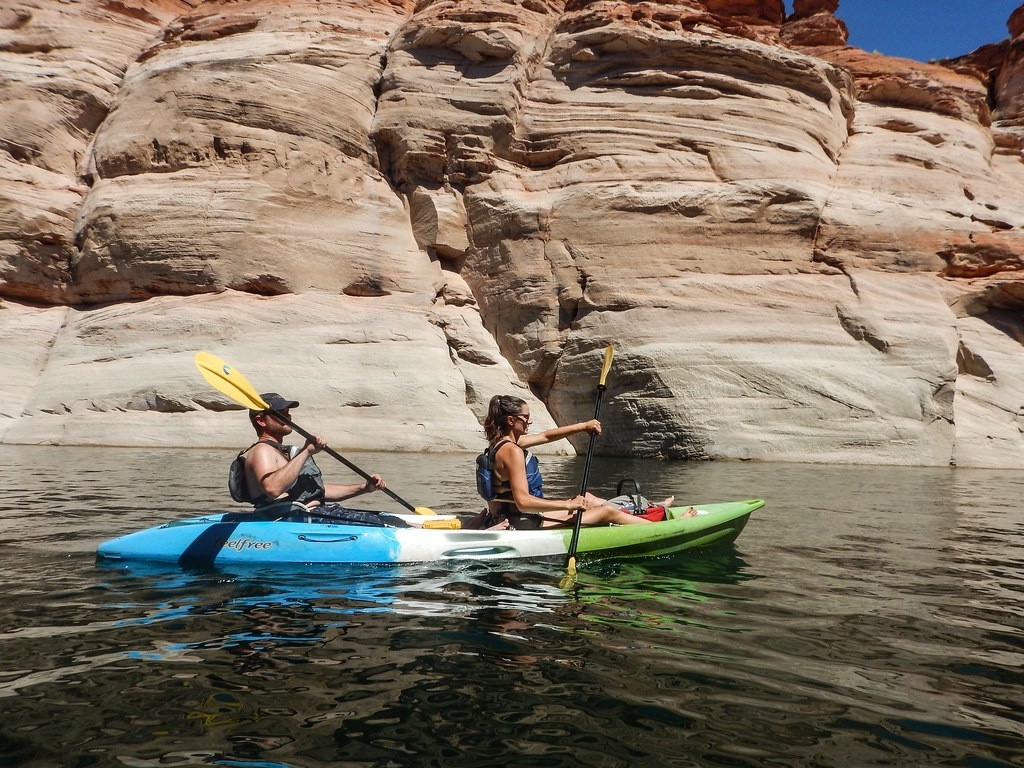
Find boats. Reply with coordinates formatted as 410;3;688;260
93;476;768;572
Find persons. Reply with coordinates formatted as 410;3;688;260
245;392;509;530
484;394;697;530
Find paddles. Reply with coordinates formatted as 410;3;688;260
195;350;438;516
566;344;615;571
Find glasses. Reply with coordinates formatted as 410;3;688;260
506;412;531;422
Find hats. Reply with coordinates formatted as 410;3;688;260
249;393;299;421
